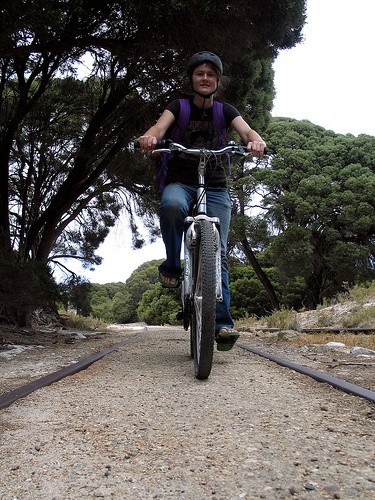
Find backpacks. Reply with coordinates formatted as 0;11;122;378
154;99;231;194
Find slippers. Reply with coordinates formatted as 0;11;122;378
158;272;179;288
216;327;240;351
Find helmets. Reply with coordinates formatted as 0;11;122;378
187;51;223;75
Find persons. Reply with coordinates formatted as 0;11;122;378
136;50;266;351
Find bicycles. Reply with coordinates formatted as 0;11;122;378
128;139;278;381
